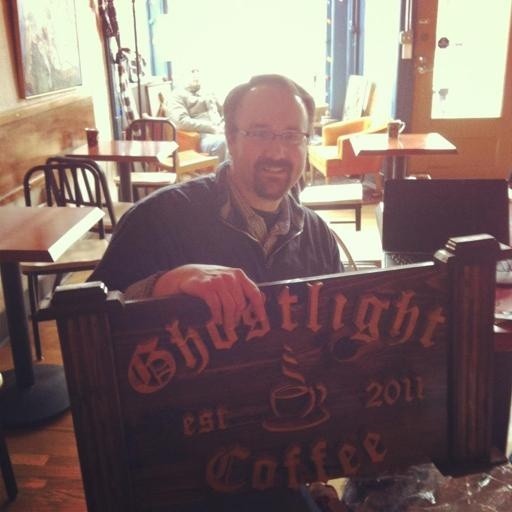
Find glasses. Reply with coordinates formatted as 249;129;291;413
238;127;311;144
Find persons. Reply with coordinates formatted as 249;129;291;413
81;74;347;511
164;76;216;135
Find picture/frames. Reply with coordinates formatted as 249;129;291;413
12;0;84;101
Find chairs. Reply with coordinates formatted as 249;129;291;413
289;73;395;231
24;156;117;361
0;437;18;501
125;75;225;204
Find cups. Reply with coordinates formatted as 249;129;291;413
387;120;406;137
388;138;404;150
85;128;98;148
